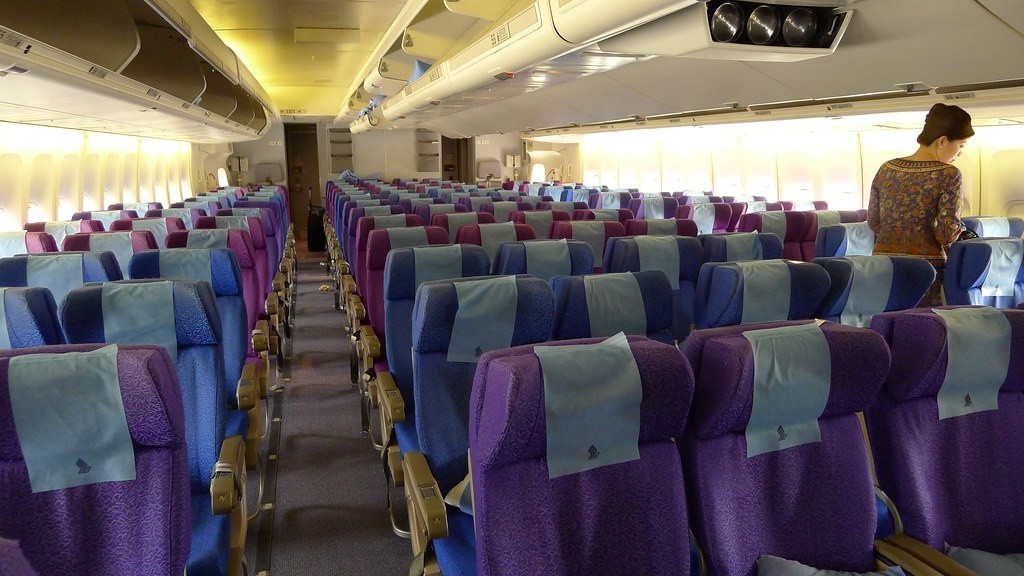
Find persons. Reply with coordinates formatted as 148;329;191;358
866;103;975;306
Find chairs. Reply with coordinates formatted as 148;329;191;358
0;178;1024;576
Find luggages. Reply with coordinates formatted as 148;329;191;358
307;187;325;252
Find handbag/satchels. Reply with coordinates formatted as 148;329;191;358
958;221;979;240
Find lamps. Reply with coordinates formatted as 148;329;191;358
701;0;855;58
